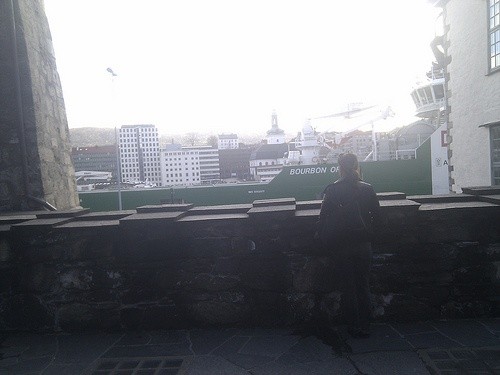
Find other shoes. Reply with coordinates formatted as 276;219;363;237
350;325;375;340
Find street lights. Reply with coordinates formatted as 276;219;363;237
106;68;122;212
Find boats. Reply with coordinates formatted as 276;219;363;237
77;58;446;211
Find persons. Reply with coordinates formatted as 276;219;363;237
317;154;382;338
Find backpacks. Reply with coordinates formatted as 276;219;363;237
332;181;375;244
